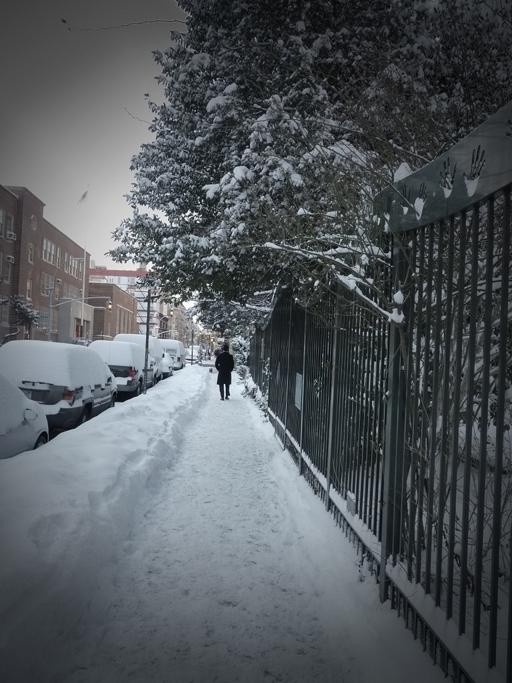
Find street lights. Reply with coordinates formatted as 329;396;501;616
47;286;114;348
169;308;175;337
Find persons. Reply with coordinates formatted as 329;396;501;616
215;344;234;400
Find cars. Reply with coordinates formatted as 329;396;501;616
1;332;214;462
